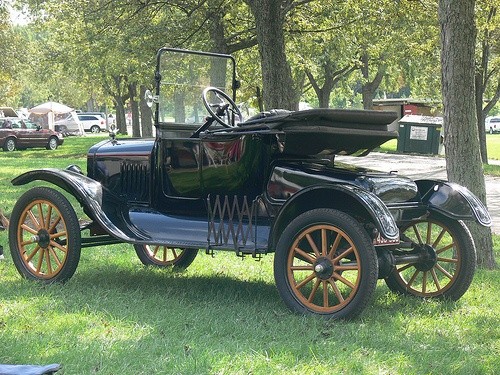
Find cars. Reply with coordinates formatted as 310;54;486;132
485;116;500;134
8;47;493;321
0;117;65;152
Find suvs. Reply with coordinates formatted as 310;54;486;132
54;112;106;134
